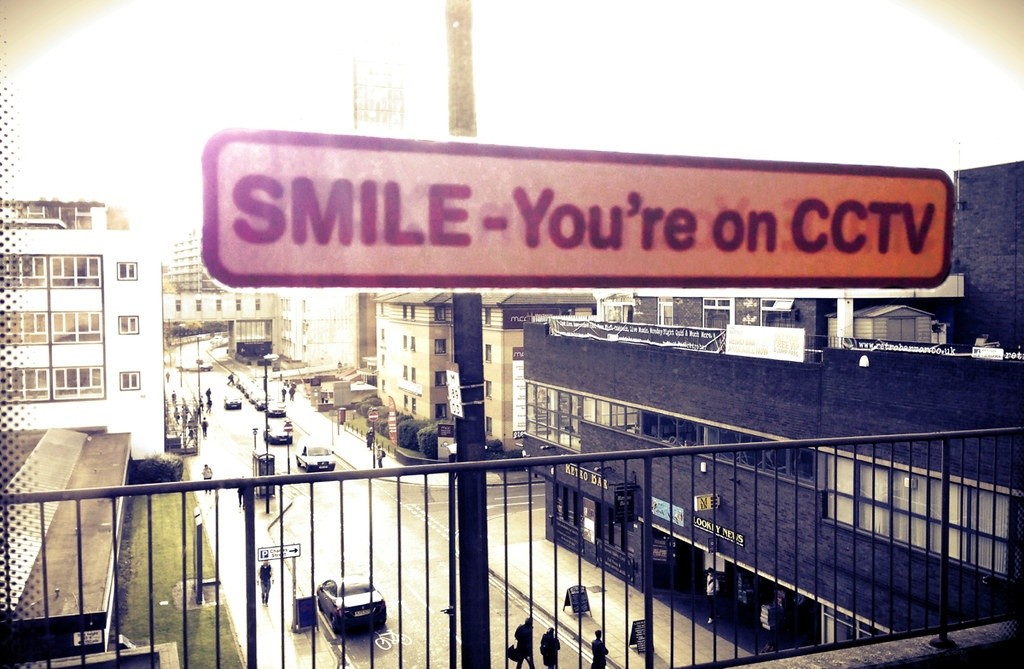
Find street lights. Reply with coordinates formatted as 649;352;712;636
178;324;186;387
262;353;280;514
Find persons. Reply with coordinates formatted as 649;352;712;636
281;386;295;402
541;628;560;669
591;630;608;669
166;372;170;383
227;372;235;385
377;444;382;468
200;388;212;414
172;390;177;404
238;476;245;510
202;464;212;495
706;568;719;623
174;405;208;439
515;618;535;669
257;560;274;607
367;427;374;451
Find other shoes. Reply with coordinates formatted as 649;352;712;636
239;503;241;507
209;491;211;495
265;600;268;606
262;597;265;604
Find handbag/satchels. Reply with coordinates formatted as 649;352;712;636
508;645;519;662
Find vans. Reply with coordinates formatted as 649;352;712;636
295;436;336;472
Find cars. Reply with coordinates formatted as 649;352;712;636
223;394;243;410
316;577;386;635
263;420;293;445
235;377;287;418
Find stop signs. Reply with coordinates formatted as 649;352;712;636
368;411;378;422
283;423;293;432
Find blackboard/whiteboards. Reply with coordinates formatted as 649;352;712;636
628;619;654;652
293;597;320;629
564;585;590;613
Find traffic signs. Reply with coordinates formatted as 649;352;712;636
257;543;302;561
